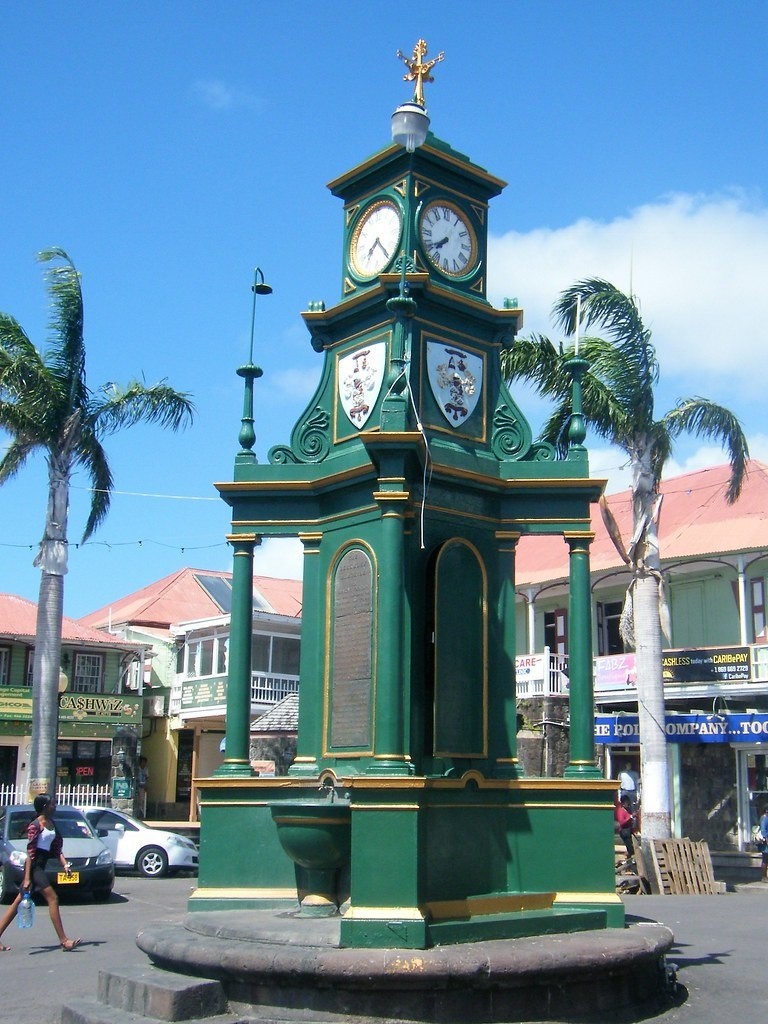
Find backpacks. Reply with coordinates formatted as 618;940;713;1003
751;825;763;845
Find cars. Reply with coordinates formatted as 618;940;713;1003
0;804;116;904
71;805;200;878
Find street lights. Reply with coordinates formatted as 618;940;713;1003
565;293;603;780
216;266;273;774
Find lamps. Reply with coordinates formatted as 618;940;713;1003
390;92;432;153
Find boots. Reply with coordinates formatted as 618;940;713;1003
761;864;768;883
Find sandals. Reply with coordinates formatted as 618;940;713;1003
0;942;11;952
61;938;82;952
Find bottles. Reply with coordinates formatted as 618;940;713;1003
17;888;35;929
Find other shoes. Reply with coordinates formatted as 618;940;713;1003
632;830;641;838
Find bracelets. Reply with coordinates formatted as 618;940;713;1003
64;864;70;868
631;815;634;819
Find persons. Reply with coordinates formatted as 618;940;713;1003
618;761;640;812
617;795;636;858
0;793;84;952
138;757;152;785
760;806;768;883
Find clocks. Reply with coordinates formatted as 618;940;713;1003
355;204;399;275
422;204;472;274
